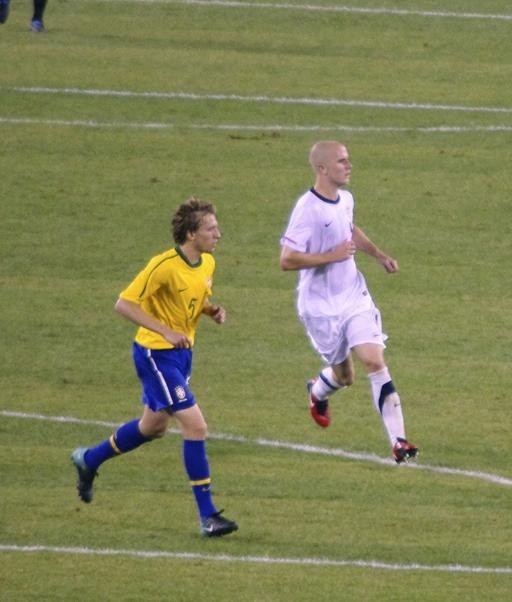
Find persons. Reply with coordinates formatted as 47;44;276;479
0;0;49;33
68;198;239;538
279;139;419;464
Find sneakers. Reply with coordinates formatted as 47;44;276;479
31;16;44;32
200;509;238;538
70;447;99;503
306;378;331;427
393;437;418;465
